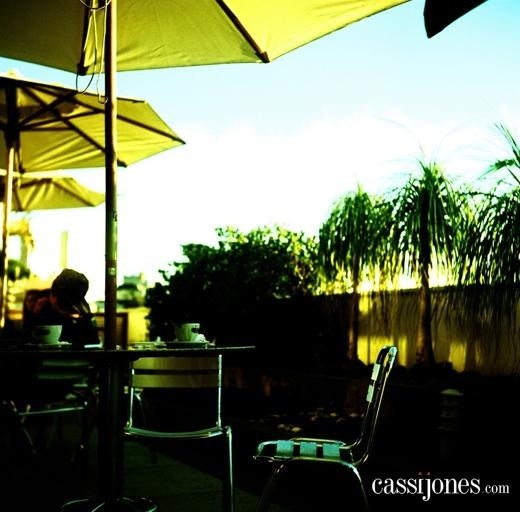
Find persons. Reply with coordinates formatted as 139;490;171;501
23;270;100;344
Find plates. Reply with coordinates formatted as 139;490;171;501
164;340;209;348
27;343;73;348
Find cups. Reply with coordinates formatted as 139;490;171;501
40;325;62;343
175;323;200;342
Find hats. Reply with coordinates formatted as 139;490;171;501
52;268;88;302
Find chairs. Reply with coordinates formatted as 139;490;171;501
0;353;233;507
252;346;397;512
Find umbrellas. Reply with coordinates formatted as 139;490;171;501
0;169;104;211
0;0;488;512
0;70;186;329
